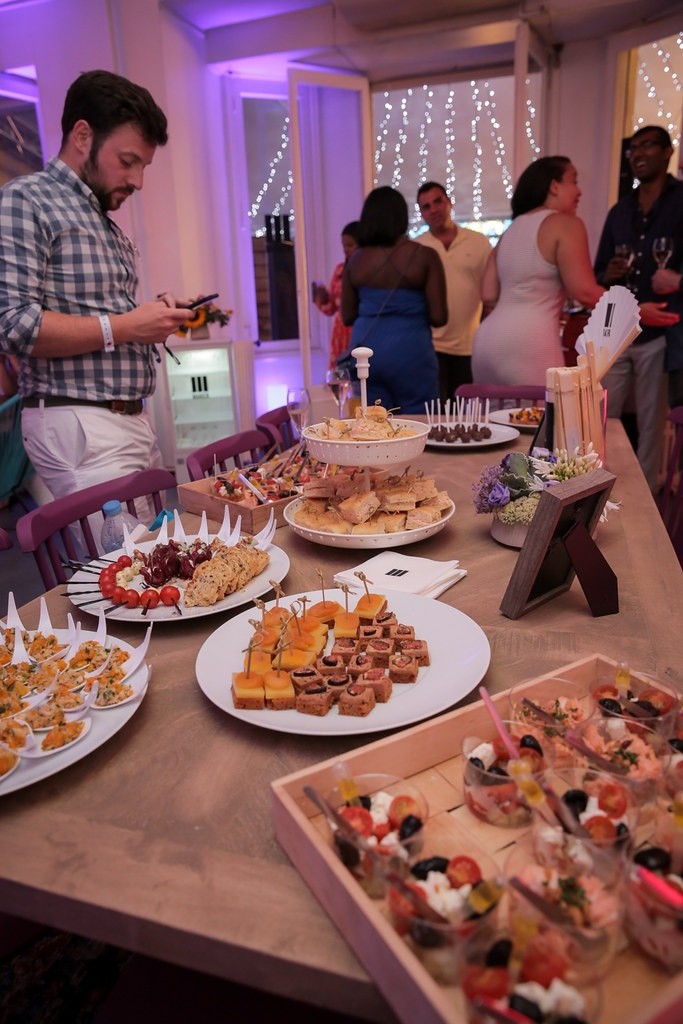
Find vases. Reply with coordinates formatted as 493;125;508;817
189;323;211;341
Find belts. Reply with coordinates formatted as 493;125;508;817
22;396;143;415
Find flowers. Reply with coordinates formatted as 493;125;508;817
172;296;234;338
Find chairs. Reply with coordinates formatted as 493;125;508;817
455;383;546;415
0;393;298;591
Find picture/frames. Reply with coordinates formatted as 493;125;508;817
499;466;621;622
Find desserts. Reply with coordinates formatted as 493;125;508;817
230;594;430;719
509;408;545;425
428;423;492;443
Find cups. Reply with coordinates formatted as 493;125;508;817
324;669;683;1023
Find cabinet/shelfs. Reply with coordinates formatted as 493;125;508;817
161;341;245;469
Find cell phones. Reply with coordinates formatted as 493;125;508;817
187;293;219;310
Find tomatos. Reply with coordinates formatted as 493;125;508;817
97;556;180;607
333;683;683;1024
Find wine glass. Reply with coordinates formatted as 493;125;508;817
605;243;638;293
287;388;311;443
325;368;352;420
650;237;672;270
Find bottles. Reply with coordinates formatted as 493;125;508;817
101;499;142;554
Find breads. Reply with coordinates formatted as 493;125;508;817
314;403;415;442
185;543;270;607
291;474;452;535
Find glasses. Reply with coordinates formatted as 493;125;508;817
624;139;661;160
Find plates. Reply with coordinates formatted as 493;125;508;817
0;628;149;797
66;534;290;622
489;407;545;428
194;587;492;735
425;422;521;447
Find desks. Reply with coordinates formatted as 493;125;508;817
0;414;683;1024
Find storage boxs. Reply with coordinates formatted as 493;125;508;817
270;652;683;1024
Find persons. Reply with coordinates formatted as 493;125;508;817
592;124;683;499
0;69;198;563
341;186;449;416
471;153;679;411
312;181;494;416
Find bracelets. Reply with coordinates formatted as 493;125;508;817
99;313;119;353
